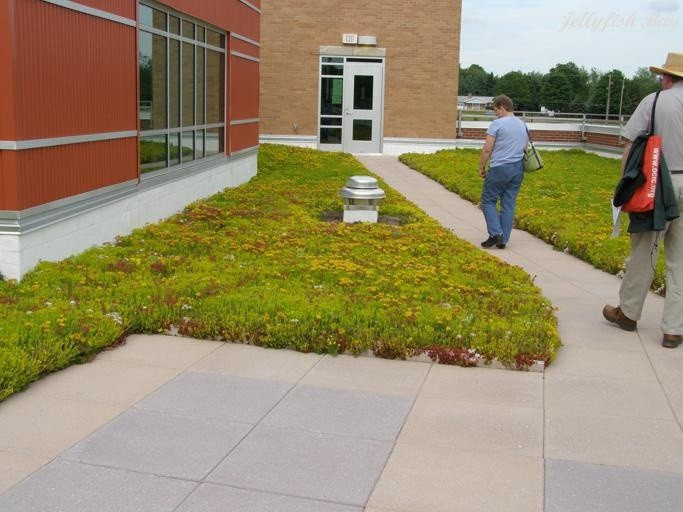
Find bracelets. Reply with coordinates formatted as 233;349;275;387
480;166;485;169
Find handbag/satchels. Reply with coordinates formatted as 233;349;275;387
523;124;544;174
620;90;663;213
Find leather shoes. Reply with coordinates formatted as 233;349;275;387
661;333;683;348
603;304;637;331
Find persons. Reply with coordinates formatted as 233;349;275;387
556;108;561;118
600;50;683;348
478;93;529;250
320;99;331;142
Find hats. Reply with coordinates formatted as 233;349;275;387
649;51;683;80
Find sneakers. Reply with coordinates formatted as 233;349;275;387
480;234;502;247
496;242;507;249
